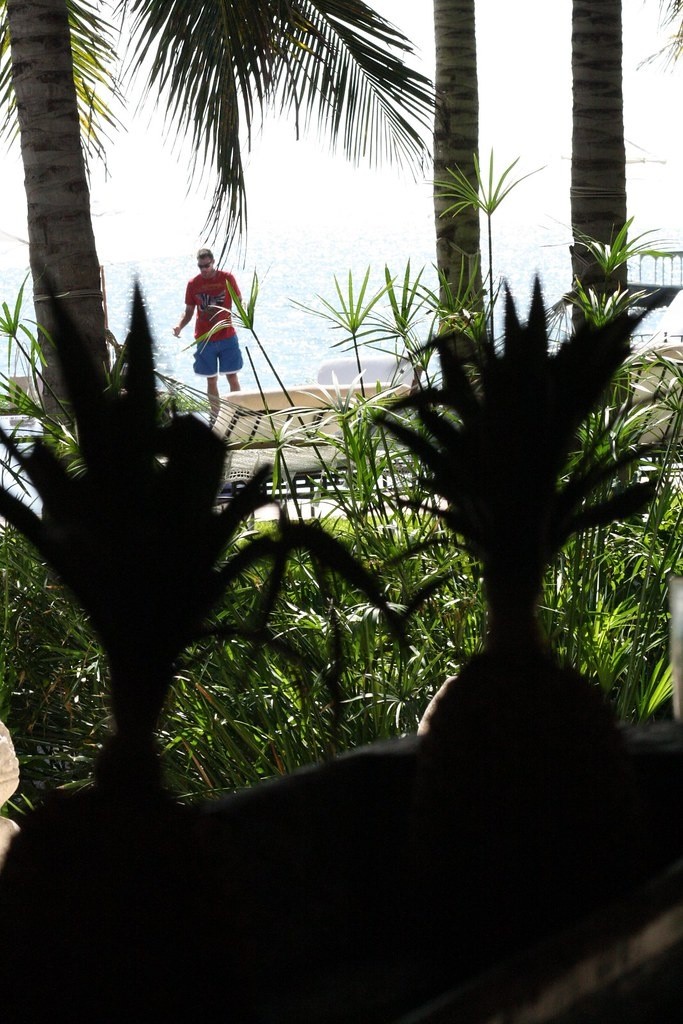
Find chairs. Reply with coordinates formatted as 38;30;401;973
208;342;683;521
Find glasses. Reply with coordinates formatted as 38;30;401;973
198;260;212;268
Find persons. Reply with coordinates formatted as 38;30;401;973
173;248;243;426
